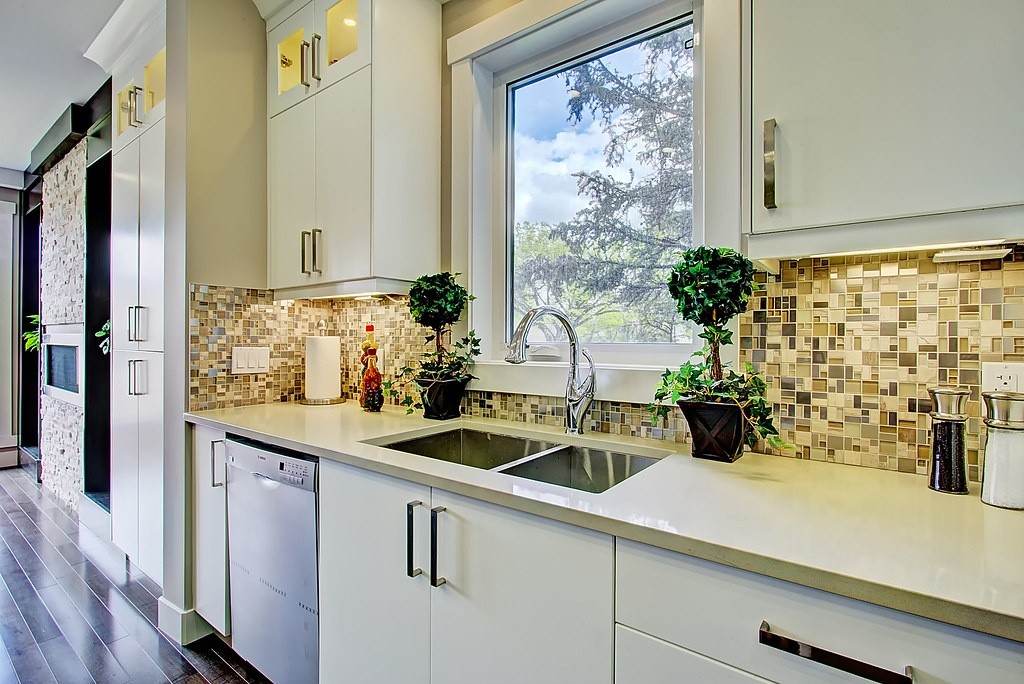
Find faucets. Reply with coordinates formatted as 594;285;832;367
505;305;601;434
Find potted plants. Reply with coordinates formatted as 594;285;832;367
379;271;482;422
642;244;796;466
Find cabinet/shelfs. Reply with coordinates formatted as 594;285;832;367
190;424;230;644
319;455;615;684
736;0;1024;262
264;0;445;300
80;0;166;593
612;537;1024;684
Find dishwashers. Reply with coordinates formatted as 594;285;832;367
225;436;319;684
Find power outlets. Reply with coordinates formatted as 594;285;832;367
375;349;385;375
980;361;1024;419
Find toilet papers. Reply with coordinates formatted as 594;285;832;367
304;336;341;399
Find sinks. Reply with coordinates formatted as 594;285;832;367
494;442;675;495
376;418;565;472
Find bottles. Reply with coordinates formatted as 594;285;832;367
981;391;1024;510
928;387;973;495
364;348;384;412
360;325;378;407
